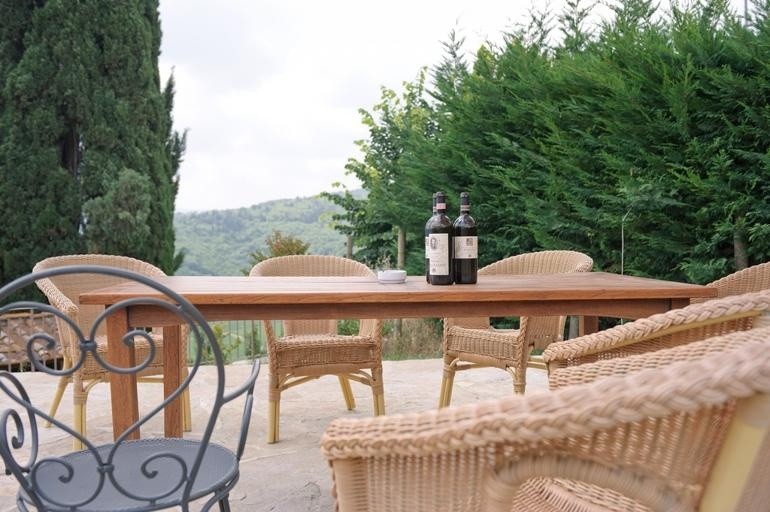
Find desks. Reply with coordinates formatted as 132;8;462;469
80;270;719;444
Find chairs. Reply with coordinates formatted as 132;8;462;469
0;265;259;512
543;289;770;371
322;328;770;512
438;250;595;412
31;254;191;452
251;255;385;444
689;260;770;304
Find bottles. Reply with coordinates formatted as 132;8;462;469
454;192;478;285
424;191;454;285
